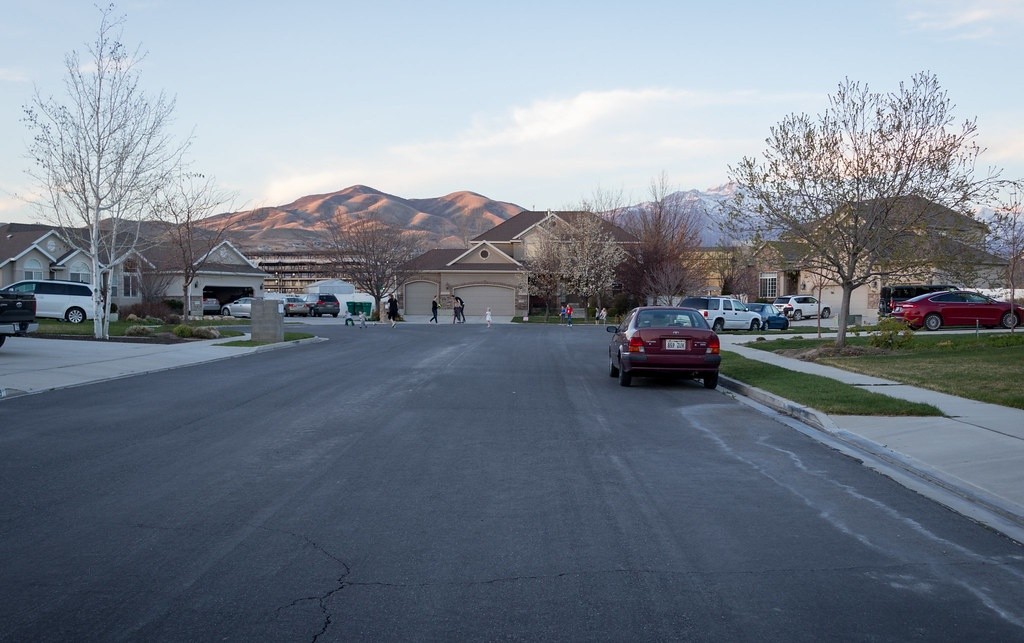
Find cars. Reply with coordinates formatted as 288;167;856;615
282;298;310;317
607;306;721;390
744;303;789;331
203;290;221;314
221;298;256;317
891;290;1024;331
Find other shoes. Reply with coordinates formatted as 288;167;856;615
392;323;396;328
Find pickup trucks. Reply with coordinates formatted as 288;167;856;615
0;290;39;347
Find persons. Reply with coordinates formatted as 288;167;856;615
452;295;465;324
486;308;492;328
345;311;355;326
558;303;573;327
429;296;440;325
594;307;608;325
387;294;398;328
359;311;368;328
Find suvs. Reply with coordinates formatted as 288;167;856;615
0;279;106;324
773;294;831;321
302;293;340;318
675;295;763;331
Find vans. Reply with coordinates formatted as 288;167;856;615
878;285;960;318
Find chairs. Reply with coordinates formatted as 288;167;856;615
666;322;683;327
638;320;650;327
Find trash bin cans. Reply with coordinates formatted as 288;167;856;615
354;302;372;317
347;302;355;315
